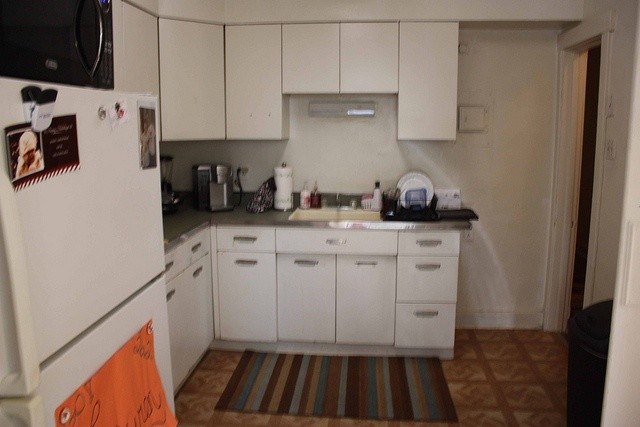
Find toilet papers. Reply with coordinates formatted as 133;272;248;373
273;166;294;209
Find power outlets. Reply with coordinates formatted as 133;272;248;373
463;229;474;241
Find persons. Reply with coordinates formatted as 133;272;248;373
145;110;156;165
140;121;150;167
17;132;45;178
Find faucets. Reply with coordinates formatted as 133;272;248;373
336;193;342;208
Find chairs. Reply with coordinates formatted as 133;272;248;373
226;25;289;140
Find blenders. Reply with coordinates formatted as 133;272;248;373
160;154;179;216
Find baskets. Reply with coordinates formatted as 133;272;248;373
395;195;438;218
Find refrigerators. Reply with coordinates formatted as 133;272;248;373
1;77;176;426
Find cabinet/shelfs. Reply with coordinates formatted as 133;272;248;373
123;2;158;93
398;22;458;140
396;231;461;361
215;227;276;353
283;22;399;94
164;226;214;396
276;226;395;356
157;16;227;140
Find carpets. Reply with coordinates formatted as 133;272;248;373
215;349;458;421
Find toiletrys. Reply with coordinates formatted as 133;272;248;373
310;180;321;208
300;181;310;210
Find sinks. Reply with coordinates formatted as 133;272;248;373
288;206;382;222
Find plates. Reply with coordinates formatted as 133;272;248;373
396;173;434;210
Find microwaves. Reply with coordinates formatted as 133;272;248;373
0;0;115;90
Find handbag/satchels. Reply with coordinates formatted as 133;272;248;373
247;178;276;213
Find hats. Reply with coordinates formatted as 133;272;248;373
19;130;36;158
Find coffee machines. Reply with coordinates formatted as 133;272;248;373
194;163;234;212
